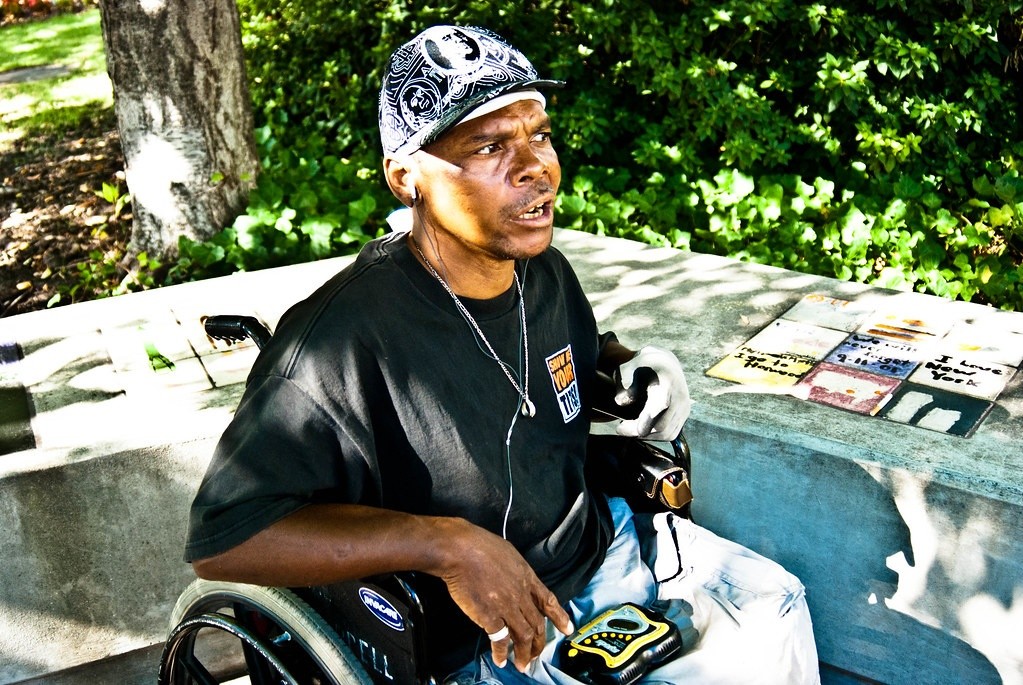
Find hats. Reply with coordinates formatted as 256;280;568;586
377;25;566;158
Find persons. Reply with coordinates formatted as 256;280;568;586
181;26;821;685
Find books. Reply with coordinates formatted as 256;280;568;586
707;291;1023;438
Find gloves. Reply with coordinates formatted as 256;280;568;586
615;345;692;441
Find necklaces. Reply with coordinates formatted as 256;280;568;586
412;238;535;417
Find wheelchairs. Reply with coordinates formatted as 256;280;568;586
155;315;693;685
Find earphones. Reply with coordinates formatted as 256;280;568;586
401;174;418;200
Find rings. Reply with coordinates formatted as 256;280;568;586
488;627;509;641
